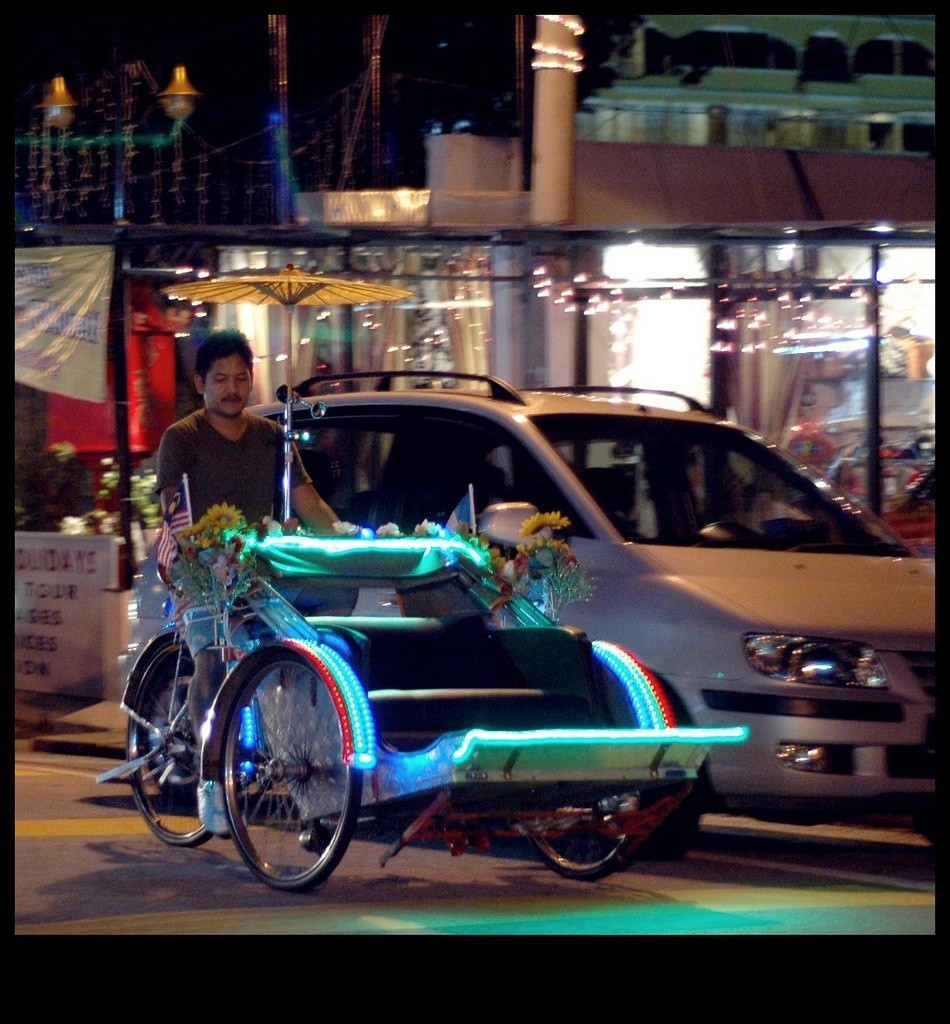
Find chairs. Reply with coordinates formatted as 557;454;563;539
433;452;633;536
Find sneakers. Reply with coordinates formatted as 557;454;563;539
197;782;232;835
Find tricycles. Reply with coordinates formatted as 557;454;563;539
95;526;749;895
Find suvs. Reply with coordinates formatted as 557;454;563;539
114;369;935;864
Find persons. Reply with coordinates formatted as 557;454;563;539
636;446;736;539
155;330;347;834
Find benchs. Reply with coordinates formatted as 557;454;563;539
300;614;591;739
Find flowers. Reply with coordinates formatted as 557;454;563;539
178;502;597;648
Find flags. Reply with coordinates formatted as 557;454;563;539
15;244;116;403
445;484;476;536
155;473;192;567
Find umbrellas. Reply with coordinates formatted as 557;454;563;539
163;261;416;404
